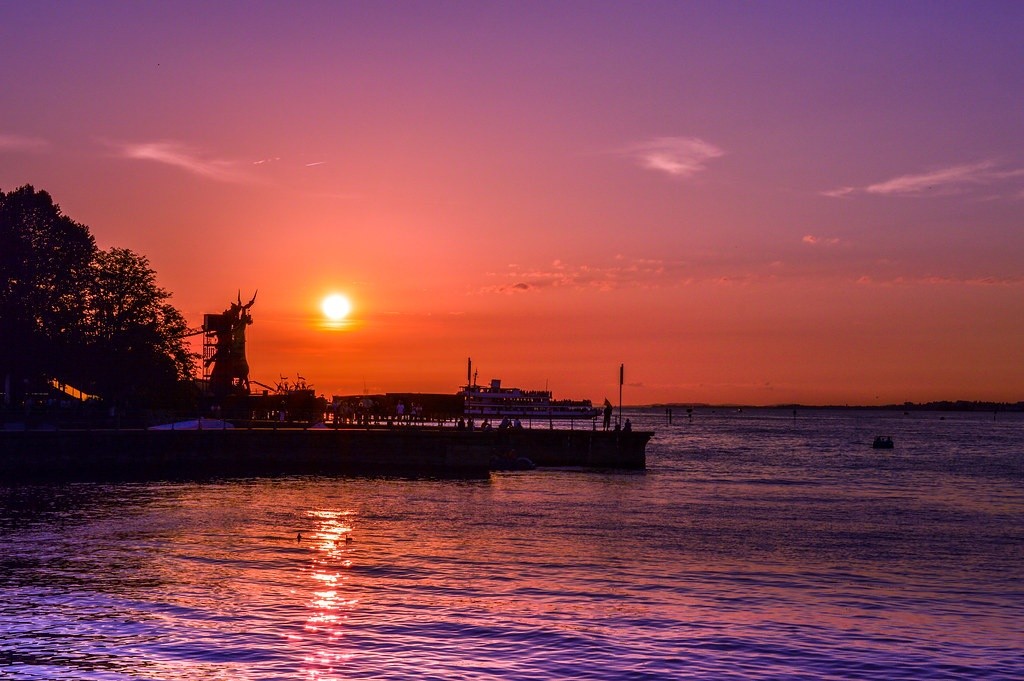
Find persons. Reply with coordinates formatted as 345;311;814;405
623;418;631;433
458;415;523;433
603;405;612;432
333;398;425;427
280;400;286;423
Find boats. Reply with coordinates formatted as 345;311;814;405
458;368;604;419
